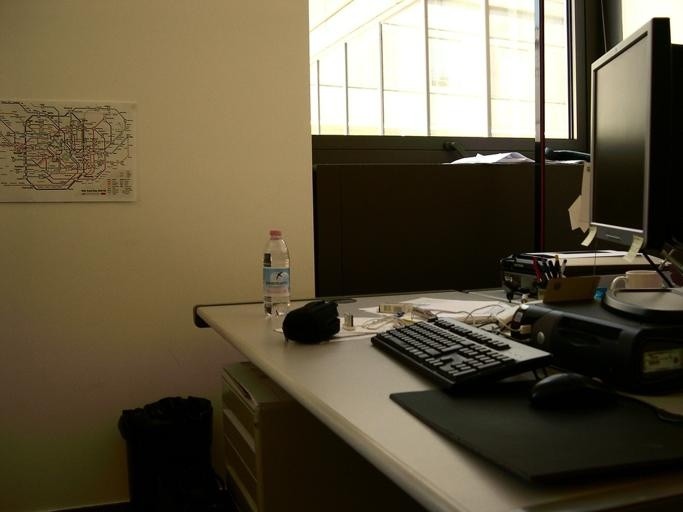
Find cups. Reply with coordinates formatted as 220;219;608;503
609;268;673;291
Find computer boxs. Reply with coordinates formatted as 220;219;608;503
512;300;683;395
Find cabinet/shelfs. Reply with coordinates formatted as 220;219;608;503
219;362;402;511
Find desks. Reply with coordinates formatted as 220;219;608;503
191;289;683;512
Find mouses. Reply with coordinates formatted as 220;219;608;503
531;373;614;410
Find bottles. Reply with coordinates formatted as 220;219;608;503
261;228;292;320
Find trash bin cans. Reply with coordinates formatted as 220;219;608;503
118;397;214;512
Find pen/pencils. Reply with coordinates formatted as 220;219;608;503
532;255;567;284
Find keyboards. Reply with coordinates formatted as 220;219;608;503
371;314;553;397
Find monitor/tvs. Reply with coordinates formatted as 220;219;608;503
589;19;682;250
